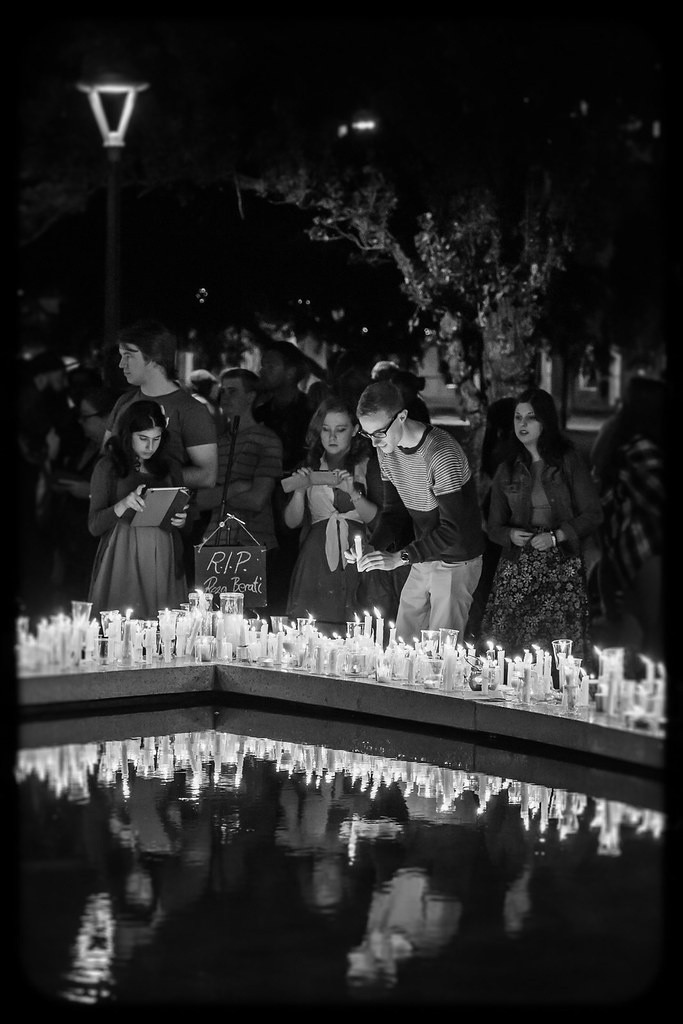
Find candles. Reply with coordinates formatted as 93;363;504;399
15;588;667;739
354;535;363;572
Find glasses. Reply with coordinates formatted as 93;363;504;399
359;408;410;438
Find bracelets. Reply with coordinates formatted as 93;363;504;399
349;490;362;503
550;530;557;549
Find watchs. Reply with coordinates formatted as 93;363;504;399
401;550;410;564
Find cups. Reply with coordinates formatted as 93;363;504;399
15;593;667;732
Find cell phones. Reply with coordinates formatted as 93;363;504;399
310;471;339;485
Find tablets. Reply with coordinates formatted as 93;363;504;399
130;487;189;531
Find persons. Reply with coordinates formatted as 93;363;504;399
284;396;384;623
21;330;140;600
344;372;484;657
195;341;335;592
591;377;665;663
99;320;218;593
87;400;190;621
479;390;604;652
